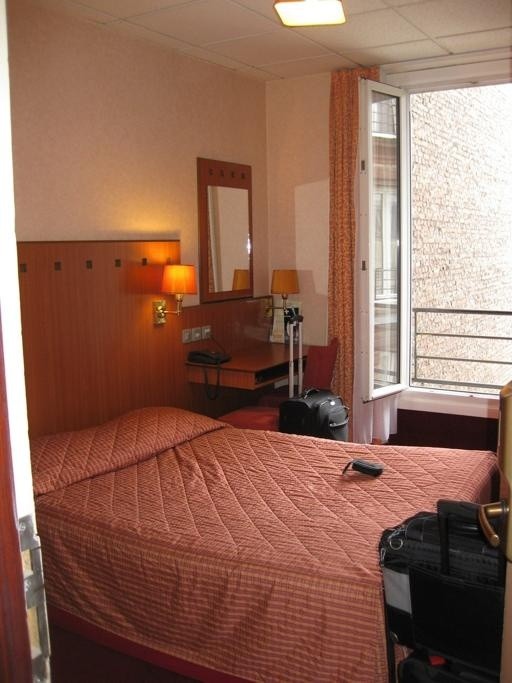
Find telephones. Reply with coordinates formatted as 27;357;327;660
188;350;231;366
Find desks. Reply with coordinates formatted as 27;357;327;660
185;343;309;391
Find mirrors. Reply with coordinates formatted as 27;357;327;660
197;157;253;304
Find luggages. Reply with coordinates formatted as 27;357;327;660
279;315;348;442
397;499;507;683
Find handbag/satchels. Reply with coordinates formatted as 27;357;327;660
379;511;507;678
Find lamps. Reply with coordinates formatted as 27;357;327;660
261;270;299;318
153;264;197;325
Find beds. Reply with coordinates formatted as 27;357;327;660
17;240;497;682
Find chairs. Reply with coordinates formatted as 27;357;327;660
217;338;339;432
